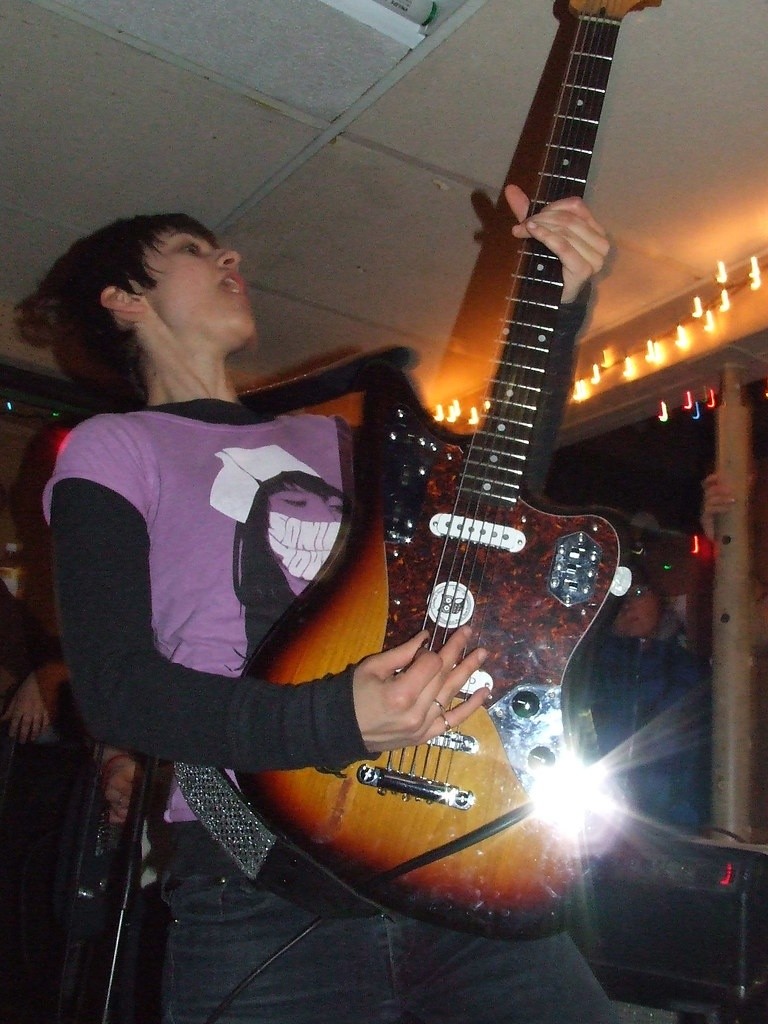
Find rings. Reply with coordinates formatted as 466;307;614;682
117;796;123;805
433;699;447;714
441;715;452;731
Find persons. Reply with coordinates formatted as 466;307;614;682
584;473;736;839
42;182;627;1024
0;577;170;1024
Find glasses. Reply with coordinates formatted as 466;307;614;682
625;588;644;602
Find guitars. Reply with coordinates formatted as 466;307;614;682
231;0;661;944
